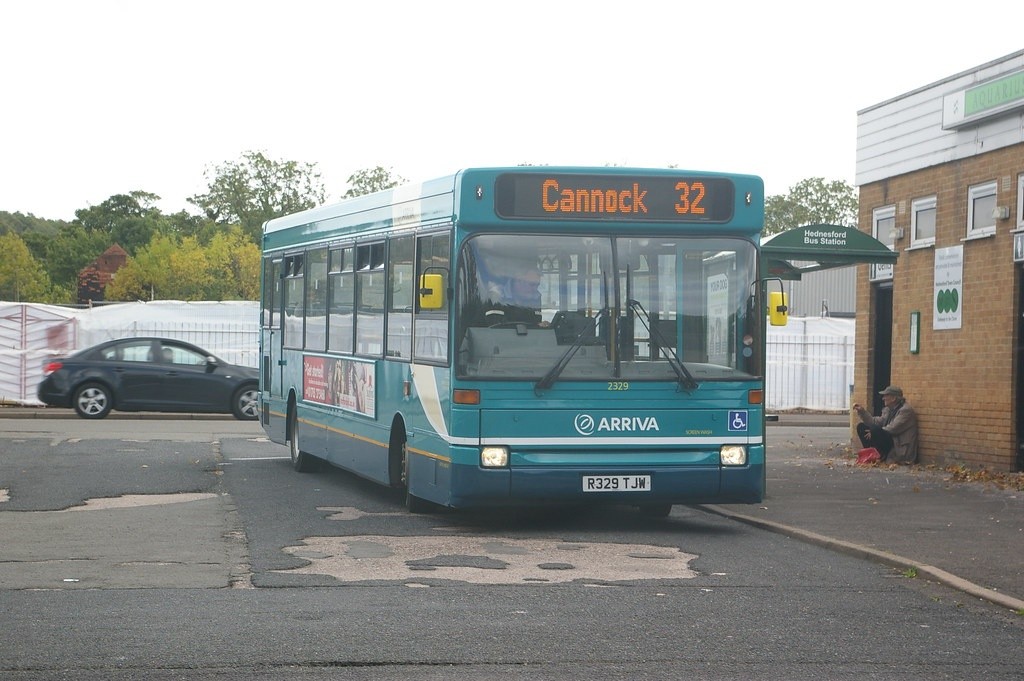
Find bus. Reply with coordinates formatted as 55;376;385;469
258;166;788;518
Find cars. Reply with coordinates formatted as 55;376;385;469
36;335;266;420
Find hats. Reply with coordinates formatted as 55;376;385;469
878;386;903;397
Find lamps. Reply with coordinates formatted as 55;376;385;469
991;205;1009;219
888;227;903;239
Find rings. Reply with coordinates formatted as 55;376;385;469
546;324;548;326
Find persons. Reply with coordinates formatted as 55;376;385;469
469;266;552;328
852;386;918;466
162;348;173;363
